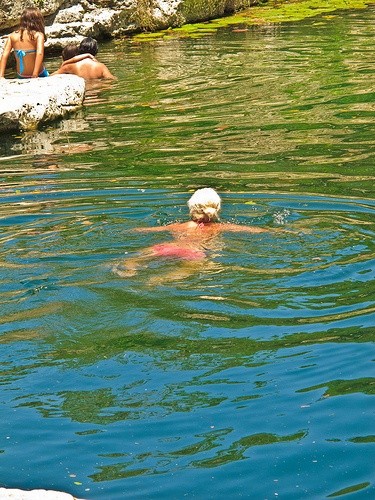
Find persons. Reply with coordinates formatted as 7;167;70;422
180;187;223;227
0;5;50;79
59;42;98;66
49;38;119;80
